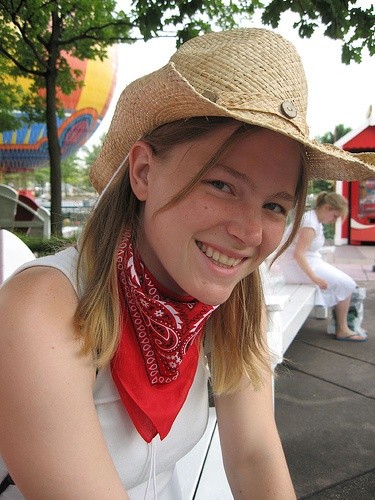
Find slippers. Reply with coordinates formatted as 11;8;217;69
335;333;369;343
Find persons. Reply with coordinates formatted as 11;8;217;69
278;191;368;342
0;28;375;500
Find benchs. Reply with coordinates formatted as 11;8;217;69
259;253;328;369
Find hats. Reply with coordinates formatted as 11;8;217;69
88;27;375;183
